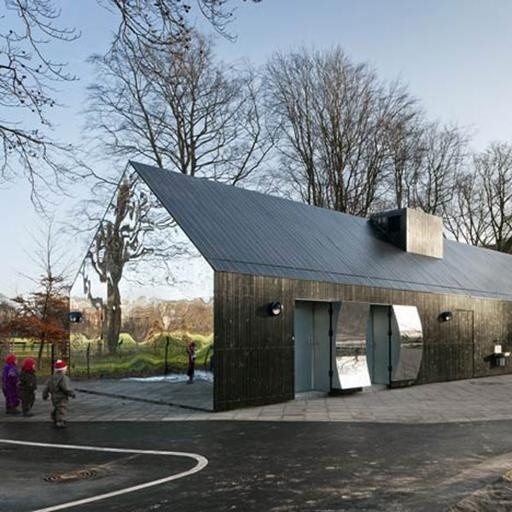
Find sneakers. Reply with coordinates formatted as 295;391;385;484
55;420;68;429
24;411;35;416
5;407;20;414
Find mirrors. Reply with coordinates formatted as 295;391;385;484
392;305;423;381
68;160;215;410
332;302;371;388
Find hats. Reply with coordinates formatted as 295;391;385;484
4;353;16;365
21;357;37;371
52;359;67;371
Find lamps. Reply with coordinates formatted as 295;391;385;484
492;338;510;368
70;312;82;323
270;302;284;315
443;312;453;321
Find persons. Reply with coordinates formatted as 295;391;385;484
17;357;38;418
42;358;77;428
2;352;22;414
186;340;197;384
